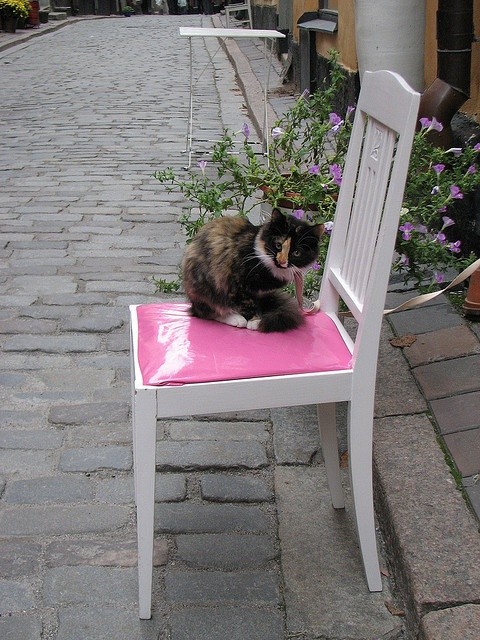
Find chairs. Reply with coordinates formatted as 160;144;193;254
224;0;253;29
127;68;433;620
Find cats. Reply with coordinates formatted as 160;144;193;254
179;208;326;333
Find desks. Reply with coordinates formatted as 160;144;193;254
180;26;286;170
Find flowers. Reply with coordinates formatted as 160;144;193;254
146;49;480;298
0;0;32;19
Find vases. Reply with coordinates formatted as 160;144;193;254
261;174;338;223
3;16;18;33
